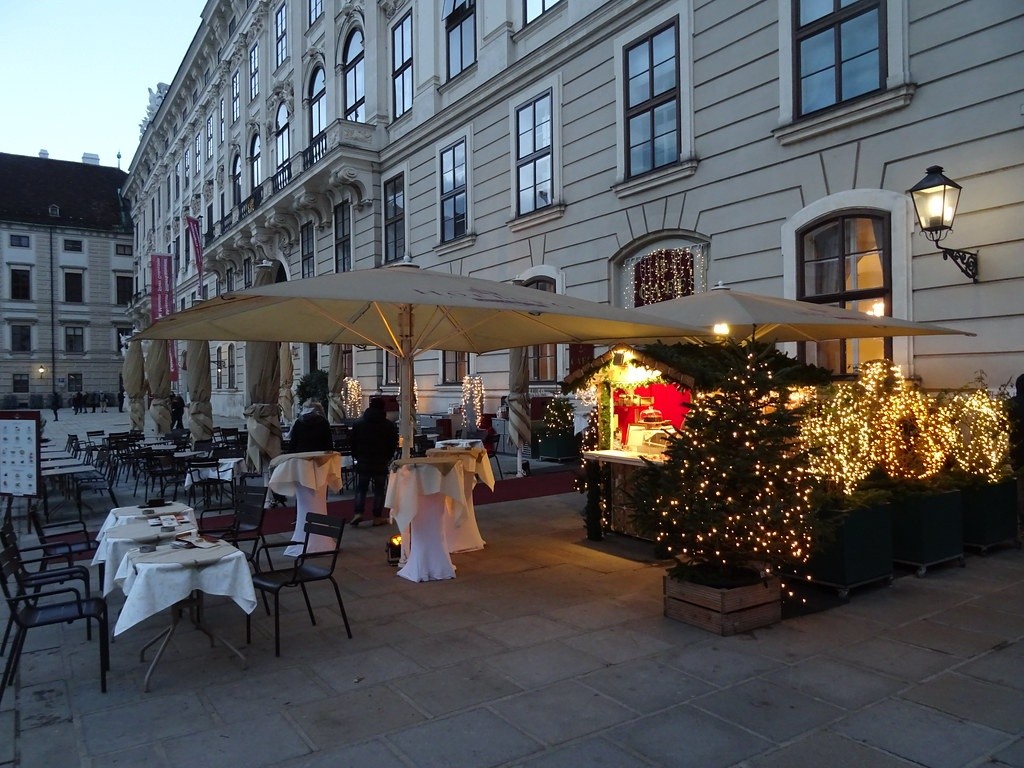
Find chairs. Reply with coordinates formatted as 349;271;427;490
0;423;503;700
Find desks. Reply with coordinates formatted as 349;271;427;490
113;539;259;694
97;501;199;548
134;438;171;476
104;518;200;619
41;447;64;491
141;444;177;486
40;452;73;496
90;434;117;465
435;439;485;450
414;433;440;450
40;465;97;523
41;457;82;513
183;458;248;507
156;450;206;499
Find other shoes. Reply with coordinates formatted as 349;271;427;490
350;514;363;525
373;517;386;526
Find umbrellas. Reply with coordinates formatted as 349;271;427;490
327;344;345;425
121;329;145;431
633;281;977;343
507;277;533;473
185;295;213;443
145;327;171;434
127;255;718;461
243;261;282;486
278;341;294;421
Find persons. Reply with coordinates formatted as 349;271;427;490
289;400;333;455
352;397;398;525
72;390;108;416
170;390;184;429
50;393;60;421
118;390;126;413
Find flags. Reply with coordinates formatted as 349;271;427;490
186;216;202;281
150;254;178;381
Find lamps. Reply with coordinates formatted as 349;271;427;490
39;365;45;379
211;360;226;372
905;165;980;285
